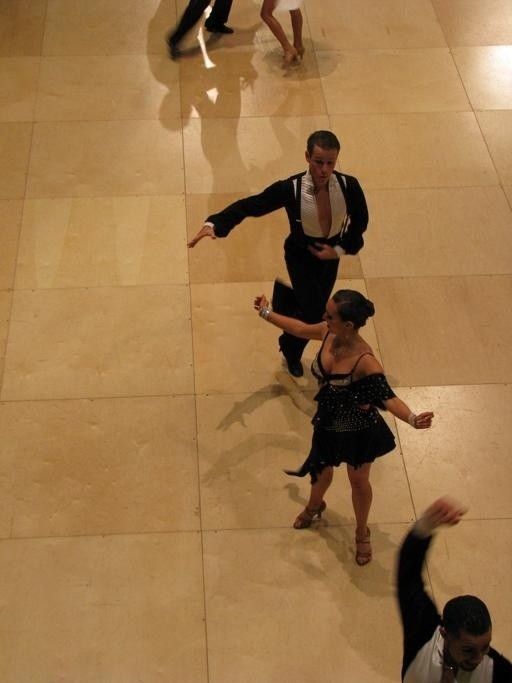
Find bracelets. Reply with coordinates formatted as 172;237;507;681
259;307;271;321
409;413;418;425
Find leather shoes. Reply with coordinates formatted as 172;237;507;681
279;334;304;377
204;17;233;34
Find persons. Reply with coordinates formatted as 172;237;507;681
396;498;512;683
253;288;433;565
188;130;368;377
165;0;233;58
260;0;304;68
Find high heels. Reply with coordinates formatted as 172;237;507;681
355;527;371;566
280;44;306;68
293;499;326;529
